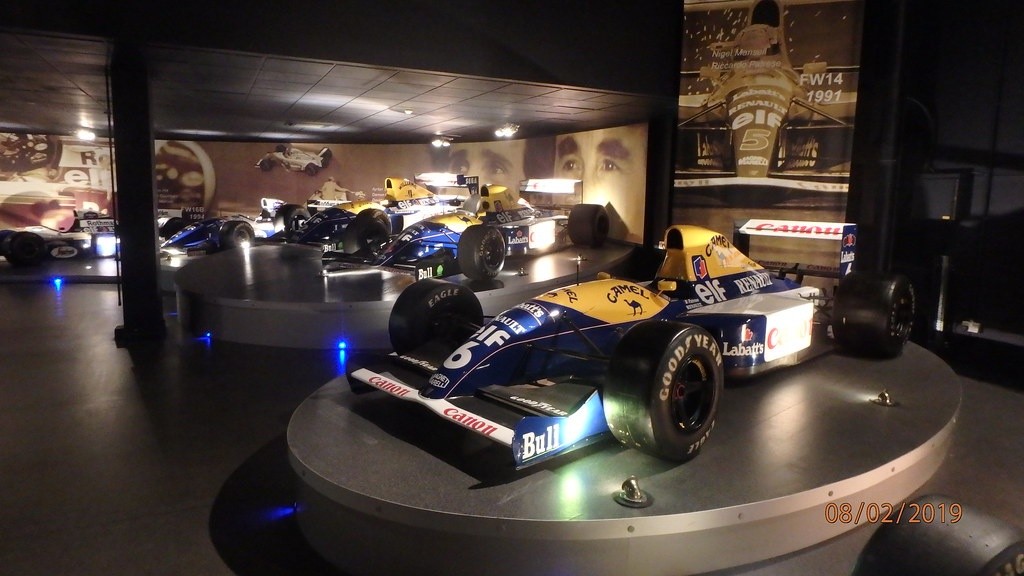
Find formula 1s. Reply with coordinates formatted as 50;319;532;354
254;145;331;177
291;171;479;249
1;207;123;266
321;178;610;284
158;198;311;257
345;215;913;473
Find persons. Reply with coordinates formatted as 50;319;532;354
440;139;533;212
302;190;318;206
549;122;648;246
321;175;350;202
309;189;326;202
